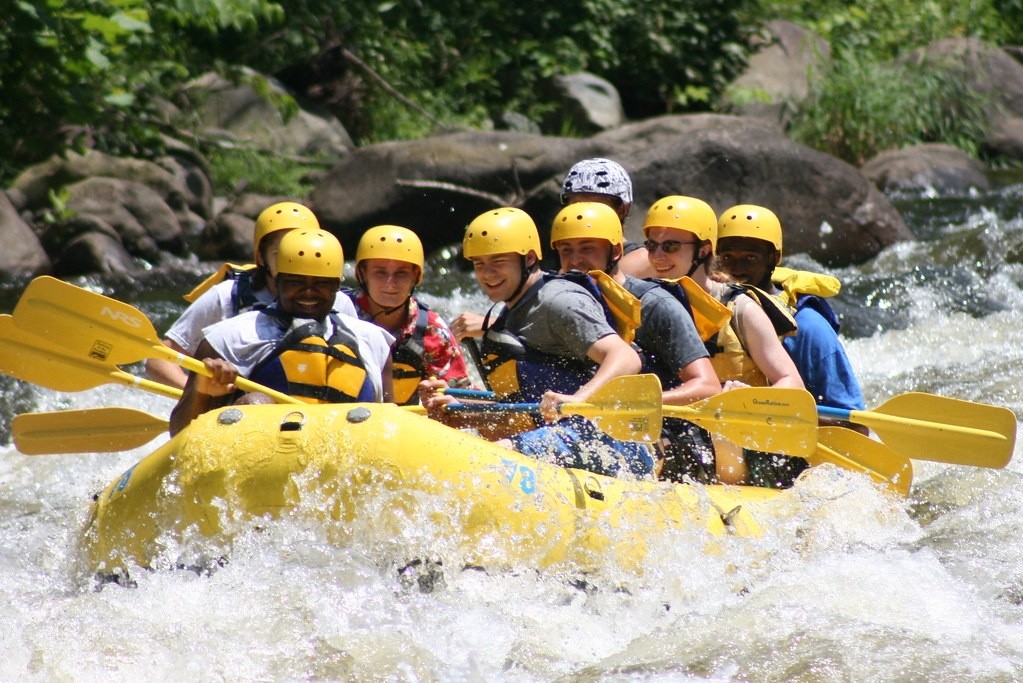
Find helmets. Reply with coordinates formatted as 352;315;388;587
254;202;320;251
643;195;718;256
550;202;624;261
463;207;543;261
561;158;633;205
276;228;344;280
355;224;424;292
718;204;782;265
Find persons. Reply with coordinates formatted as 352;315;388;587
145;158;869;489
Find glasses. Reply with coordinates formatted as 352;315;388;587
643;238;698;253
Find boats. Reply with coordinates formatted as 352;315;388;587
79;403;900;594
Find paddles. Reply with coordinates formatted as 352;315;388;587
435;386;820;458
1;310;184;400
390;372;664;446
461;333;491;392
11;408;171;454
11;274;306;406
815;424;913;497
814;392;1018;468
